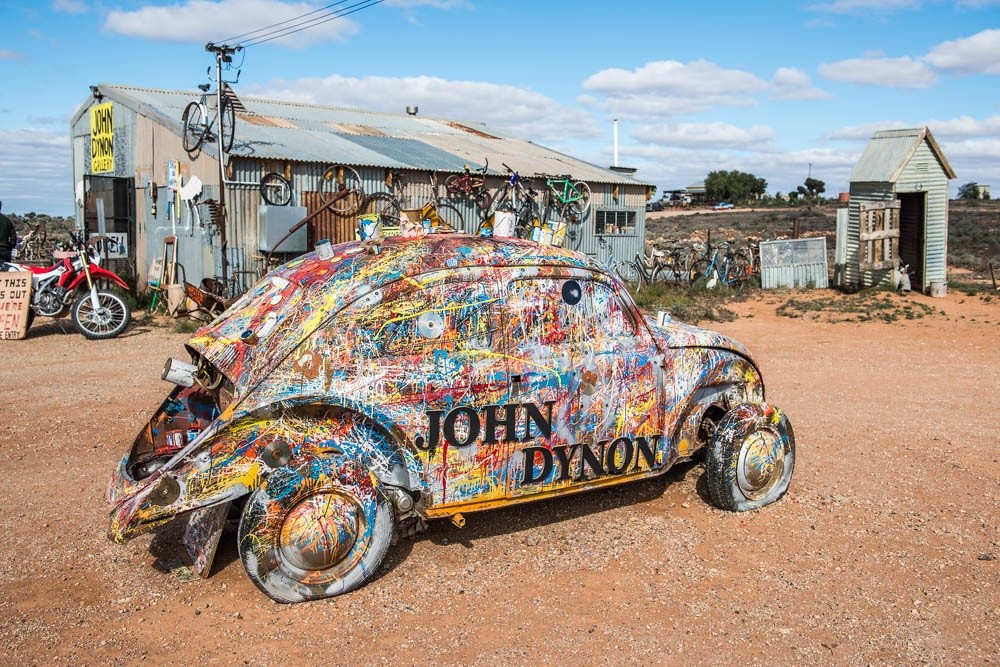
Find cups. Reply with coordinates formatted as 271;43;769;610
627;227;635;234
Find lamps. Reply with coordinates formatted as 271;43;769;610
89;83;103;102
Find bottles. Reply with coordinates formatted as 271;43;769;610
531;217;553;244
606;224;626;234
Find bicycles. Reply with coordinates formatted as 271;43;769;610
358;167;465;233
632;232;761;293
180;66;241;156
583;232;642;295
483;162;542;243
444;154;494;210
532;172;592;226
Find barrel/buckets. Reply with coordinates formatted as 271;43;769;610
314;239;335;260
399;209;423;237
930;281;947;297
357;213;384;243
494;211;516;238
657;311;671;328
186;296;203;321
160;358;199;387
547;219;568;247
167;285;185;317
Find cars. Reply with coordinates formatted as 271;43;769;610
105;232;798;603
646;202;664;212
711;202;735;211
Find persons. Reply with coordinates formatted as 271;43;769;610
0;201;16;270
896;256;911;297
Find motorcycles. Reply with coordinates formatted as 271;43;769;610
0;230;133;342
